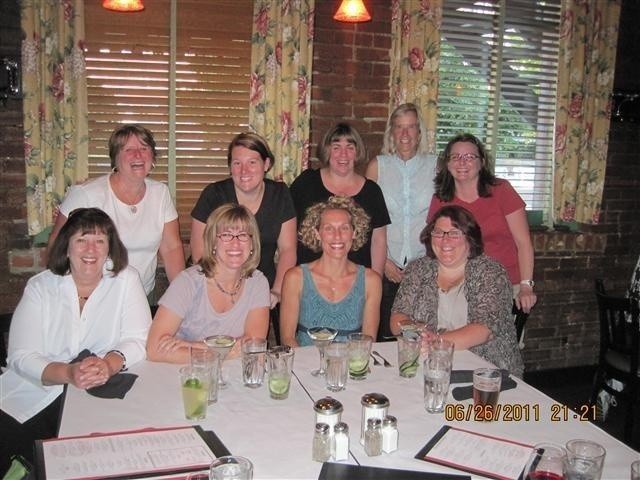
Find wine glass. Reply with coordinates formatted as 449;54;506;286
202;334;236;390
523;442;569;480
307;326;338;377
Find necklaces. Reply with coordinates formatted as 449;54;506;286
77;291;89;303
119;183;143;215
322;274;344;297
211;273;244;304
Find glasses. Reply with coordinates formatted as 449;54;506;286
448;153;482;162
216;233;252;242
430;229;466;238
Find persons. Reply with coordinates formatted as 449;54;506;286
146;203;270;365
389;204;519;378
48;123;186;297
288;122;393;278
0;207;154;480
426;132;537;346
364;101;440;340
576;256;640;423
279;194;382;347
190;133;298;345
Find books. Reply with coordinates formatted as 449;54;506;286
33;424;236;480
414;425;545;480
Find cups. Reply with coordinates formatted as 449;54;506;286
472;368;502;420
396;336;422;378
178;366;209;421
632;460;640;479
265;345;294;400
349;333;373;380
430;340;454;359
190;350;220;404
567;439;606;480
424;356;451;412
327;340;350;391
240;336;268;389
209;455;253;480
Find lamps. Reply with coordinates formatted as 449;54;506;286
102;0;146;12
333;0;372;22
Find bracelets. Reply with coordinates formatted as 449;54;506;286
105;350;129;373
439;333;444;344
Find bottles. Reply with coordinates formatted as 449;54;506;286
312;395;344;425
382;414;400;454
359;391;391;445
333;422;350;462
312;424;331;463
364;417;382;457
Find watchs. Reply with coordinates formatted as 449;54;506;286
520;279;536;286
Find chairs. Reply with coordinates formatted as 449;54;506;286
378;281;401;342
271;303;281;346
0;312;12;375
512;297;534;342
582;279;640;441
